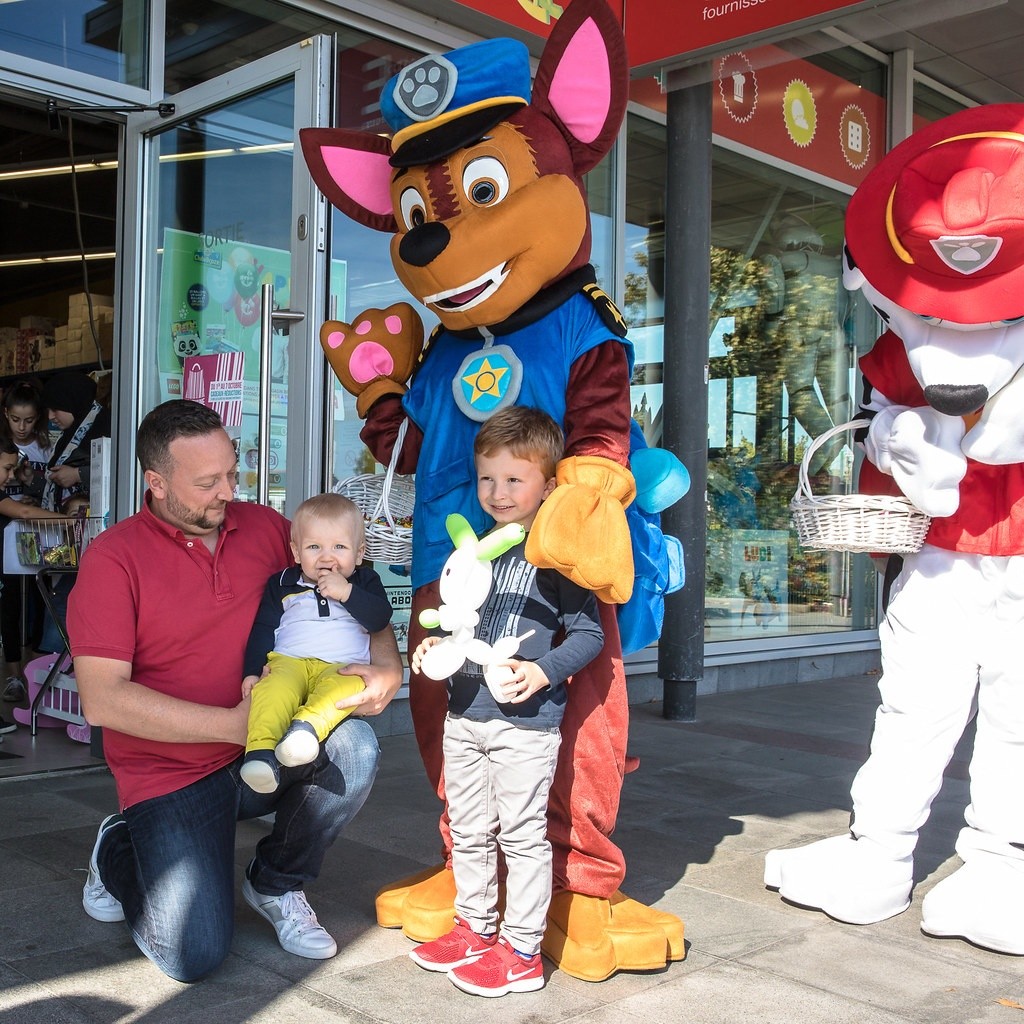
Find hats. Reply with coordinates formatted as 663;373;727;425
42;371;98;417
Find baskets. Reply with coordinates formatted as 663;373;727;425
789;418;930;555
332;416;417;565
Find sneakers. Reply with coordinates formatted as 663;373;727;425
408;916;497;973
448;936;545;998
240;855;338;960
83;813;127;922
1;675;27;701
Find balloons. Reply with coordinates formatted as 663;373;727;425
419;514;536;704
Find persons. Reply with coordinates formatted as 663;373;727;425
1;377;110;731
68;401;403;984
239;492;394;795
405;407;612;999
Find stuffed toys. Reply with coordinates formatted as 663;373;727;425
299;2;689;982
760;103;1024;955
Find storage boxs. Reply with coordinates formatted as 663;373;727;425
708;528;791;638
0;292;114;377
90;437;111;544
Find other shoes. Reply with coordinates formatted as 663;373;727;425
0;716;18;734
274;719;321;768
239;750;280;794
